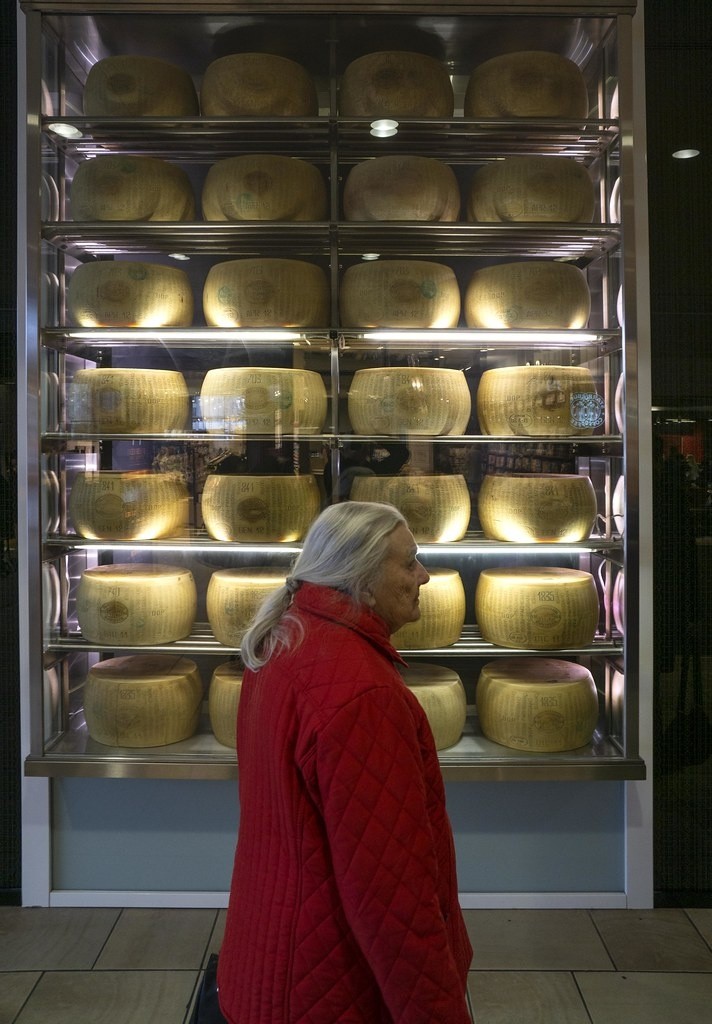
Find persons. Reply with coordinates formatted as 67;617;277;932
217;499;475;1023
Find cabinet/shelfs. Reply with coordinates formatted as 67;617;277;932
19;1;650;782
